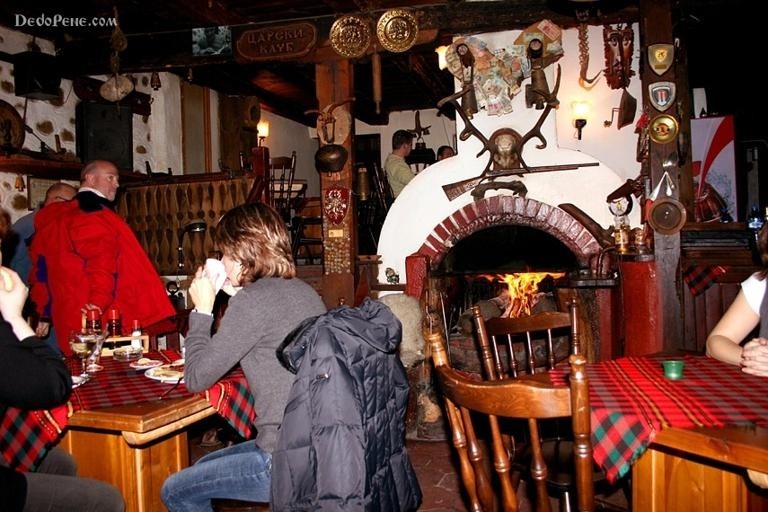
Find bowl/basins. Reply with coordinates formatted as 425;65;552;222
113;351;145;364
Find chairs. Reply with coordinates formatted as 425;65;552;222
141;147;395;267
210;297;424;512
466;294;632;512
419;325;601;512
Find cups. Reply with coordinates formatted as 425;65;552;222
663;360;684;381
203;257;227;295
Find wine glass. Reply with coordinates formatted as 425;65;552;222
71;331;106;380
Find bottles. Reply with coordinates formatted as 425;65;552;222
709;210;733;224
692;160;729;223
699;107;719;118
613;203;632;253
747;206;764;230
87;309;144;352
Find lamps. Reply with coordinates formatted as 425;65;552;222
172;216;209;278
570;100;591;141
257;120;270;147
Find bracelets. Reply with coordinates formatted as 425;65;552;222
191;309;213;317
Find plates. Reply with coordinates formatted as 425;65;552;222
130;359;187;384
71;376;87;390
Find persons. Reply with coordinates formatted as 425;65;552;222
706;219;768;378
159;202;329;511
383;130;417;201
436;146;455;162
